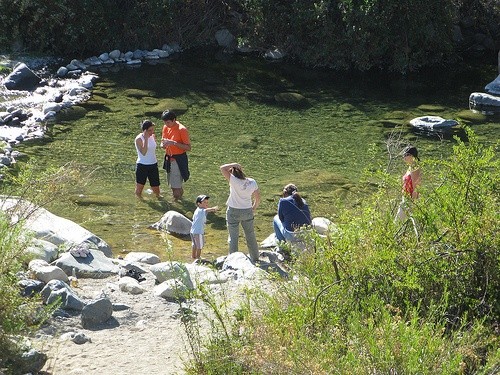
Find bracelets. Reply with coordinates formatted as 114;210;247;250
172;141;177;145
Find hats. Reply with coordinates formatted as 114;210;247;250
196;195;210;203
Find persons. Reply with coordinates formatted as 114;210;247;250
393;147;421;241
160;108;191;198
273;183;313;253
135;120;164;200
190;194;219;260
220;162;261;263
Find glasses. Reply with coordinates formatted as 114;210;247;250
404;153;413;156
